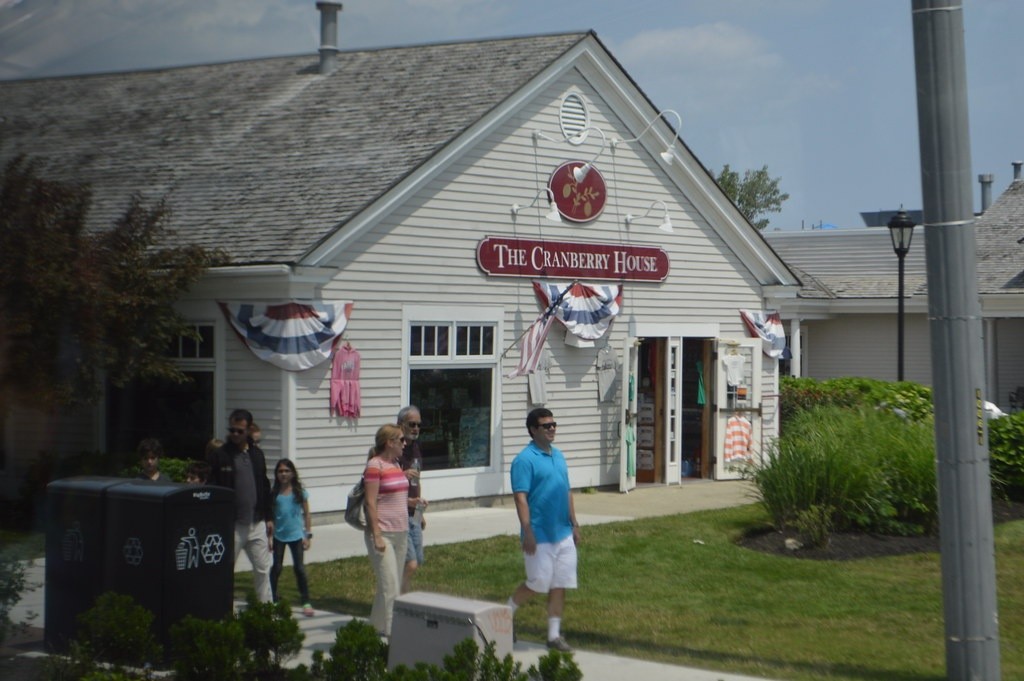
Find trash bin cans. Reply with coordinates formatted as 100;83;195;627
104;481;236;672
45;476;143;659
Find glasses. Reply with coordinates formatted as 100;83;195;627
227;425;249;435
388;436;404;443
533;422;557;429
399;421;421;428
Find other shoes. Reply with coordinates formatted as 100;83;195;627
303;604;315;616
546;637;574;653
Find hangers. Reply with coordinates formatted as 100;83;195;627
598;336;616;353
728;407;751;424
725;347;746;360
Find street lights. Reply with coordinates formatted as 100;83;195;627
886;203;917;382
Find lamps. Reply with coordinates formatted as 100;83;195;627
610;109;682;165
510;189;562;223
626;200;674;233
534;126;606;184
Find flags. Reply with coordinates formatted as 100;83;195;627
507;296;560;381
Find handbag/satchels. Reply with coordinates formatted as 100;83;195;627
345;458;382;532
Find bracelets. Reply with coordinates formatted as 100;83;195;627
305;531;312;539
573;522;579;529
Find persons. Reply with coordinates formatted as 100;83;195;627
398;406;426;592
137;438;169;482
270;458;317;617
506;408;582;653
365;424;409;647
209;409;273;604
187;462;211;485
205;437;225;461
